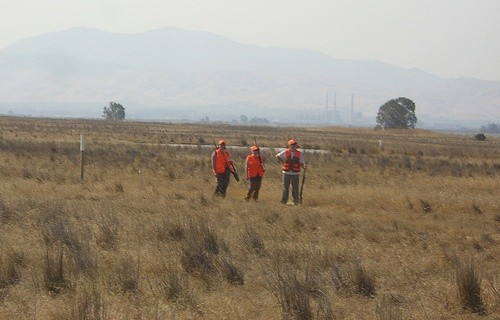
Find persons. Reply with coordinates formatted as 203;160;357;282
245;146;264;201
211;140;233;198
276;140;306;207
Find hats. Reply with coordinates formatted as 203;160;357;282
219;140;225;144
288;140;297;145
251;145;260;151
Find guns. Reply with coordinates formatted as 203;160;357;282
210;137;240;183
299;160;308;204
252;136;267;173
